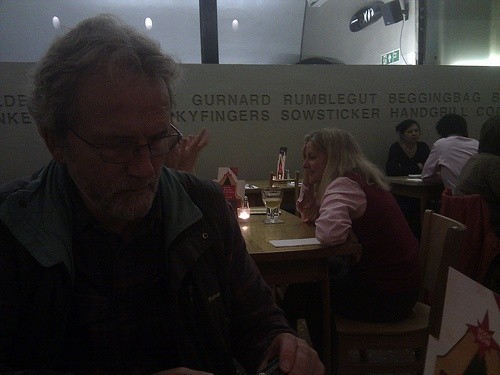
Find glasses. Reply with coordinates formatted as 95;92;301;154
68;124;184;163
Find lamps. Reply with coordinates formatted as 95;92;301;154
349;0;408;32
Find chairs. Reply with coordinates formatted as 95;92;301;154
332;209;470;375
441;187;500;294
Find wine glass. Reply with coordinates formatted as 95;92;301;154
261;187;285;224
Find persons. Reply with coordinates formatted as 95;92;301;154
284;128;421;375
385;119;430;236
0;15;327;375
454;114;500;240
162;110;209;174
421;113;479;196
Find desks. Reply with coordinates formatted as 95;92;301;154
235;206;361;375
388;175;445;224
245;180;303;216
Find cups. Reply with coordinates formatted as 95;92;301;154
237;208;250;223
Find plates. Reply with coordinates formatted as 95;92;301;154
408;174;421;178
